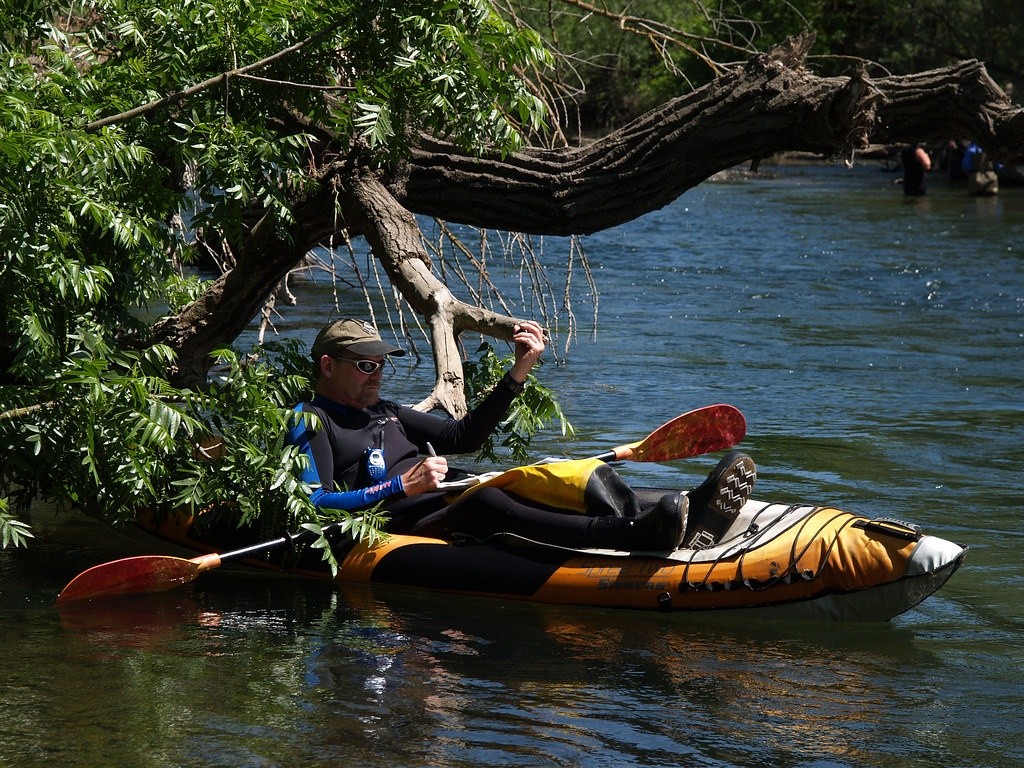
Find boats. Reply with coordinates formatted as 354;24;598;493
133;401;971;627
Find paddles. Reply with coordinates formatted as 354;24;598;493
56;401;748;606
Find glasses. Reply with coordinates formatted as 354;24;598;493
334;358;386;374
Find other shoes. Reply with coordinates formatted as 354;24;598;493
588;493;690;550
653;450;756;555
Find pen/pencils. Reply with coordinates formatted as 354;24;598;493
425;441;440;460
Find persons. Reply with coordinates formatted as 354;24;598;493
900;137;1004;198
283;318;756;552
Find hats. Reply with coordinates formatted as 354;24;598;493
309;317;406;357
